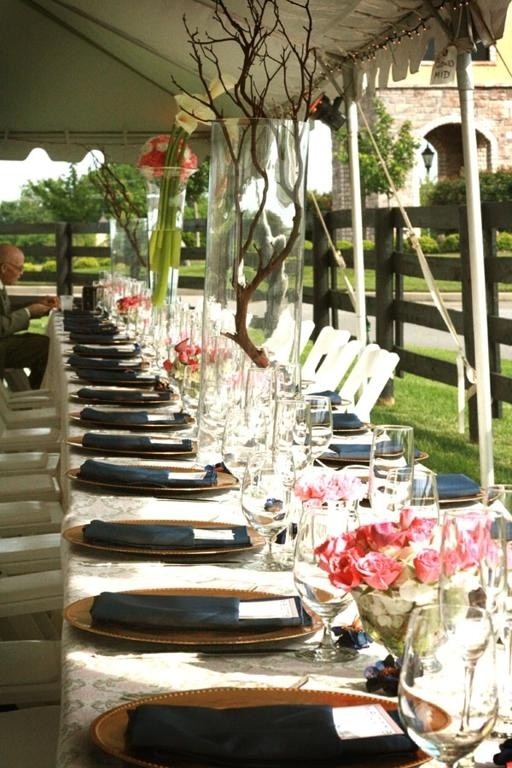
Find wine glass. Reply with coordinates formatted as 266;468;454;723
396;603;500;767
293;505;363;664
240;449;296;568
368;423;416;516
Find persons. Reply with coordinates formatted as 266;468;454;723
0;244;62;391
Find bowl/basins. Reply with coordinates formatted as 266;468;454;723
351;587;454;675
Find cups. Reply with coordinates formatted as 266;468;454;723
382;468;440;525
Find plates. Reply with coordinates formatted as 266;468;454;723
92;685;453;767
411;474;505;504
65;464;242;493
62;519;267;558
52;302;210;460
60;586;324;650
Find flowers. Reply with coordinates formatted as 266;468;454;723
141;72;233;306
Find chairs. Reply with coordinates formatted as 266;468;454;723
0;368;64;768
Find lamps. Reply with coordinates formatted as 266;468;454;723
309;91;346;131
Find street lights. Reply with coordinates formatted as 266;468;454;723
423;145;435;186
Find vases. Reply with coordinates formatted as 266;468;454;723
110;217;150;318
143;167;199;362
196;118;309;475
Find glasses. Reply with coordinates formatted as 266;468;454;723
1;262;23;272
